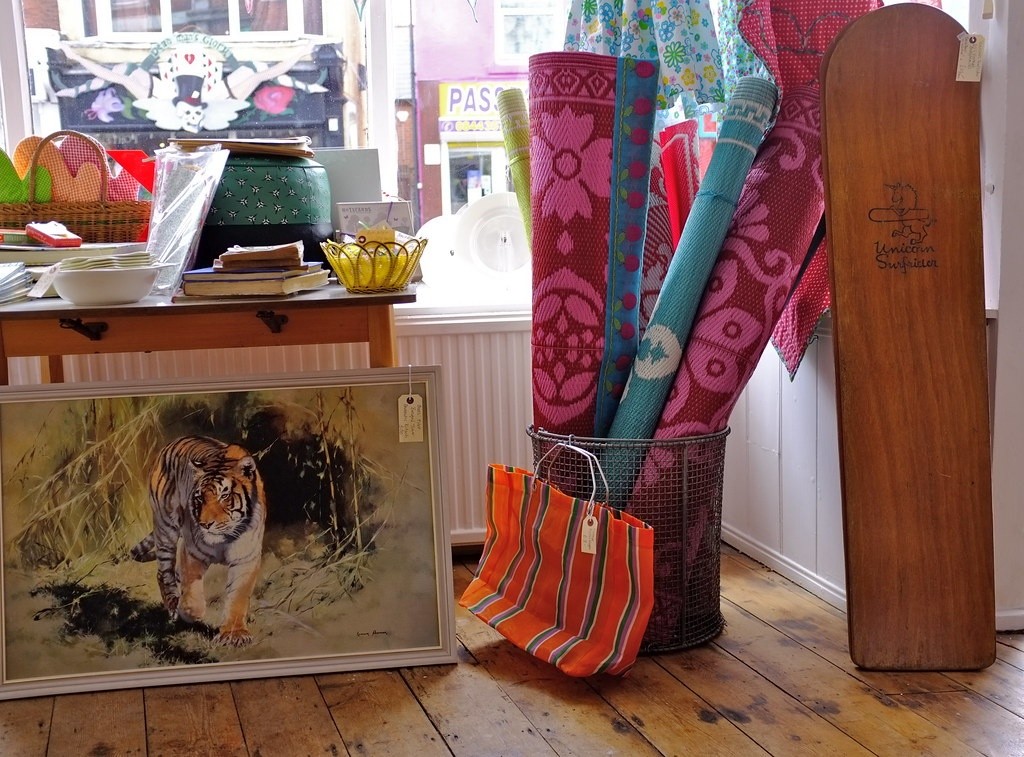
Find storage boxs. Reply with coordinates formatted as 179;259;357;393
310;147;382;228
337;202;422;283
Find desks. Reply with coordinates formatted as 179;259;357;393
0;276;418;386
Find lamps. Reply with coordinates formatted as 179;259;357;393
397;110;409;122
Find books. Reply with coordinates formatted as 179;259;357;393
0;262;37;306
172;240;330;303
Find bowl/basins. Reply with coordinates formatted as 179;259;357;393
53;264;179;306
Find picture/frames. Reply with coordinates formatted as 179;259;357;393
0;365;458;700
